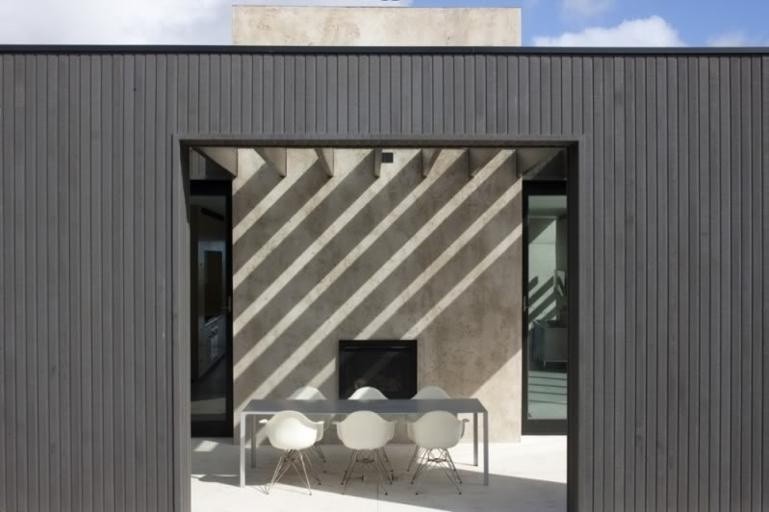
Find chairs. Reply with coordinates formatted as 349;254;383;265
240;387;488;496
